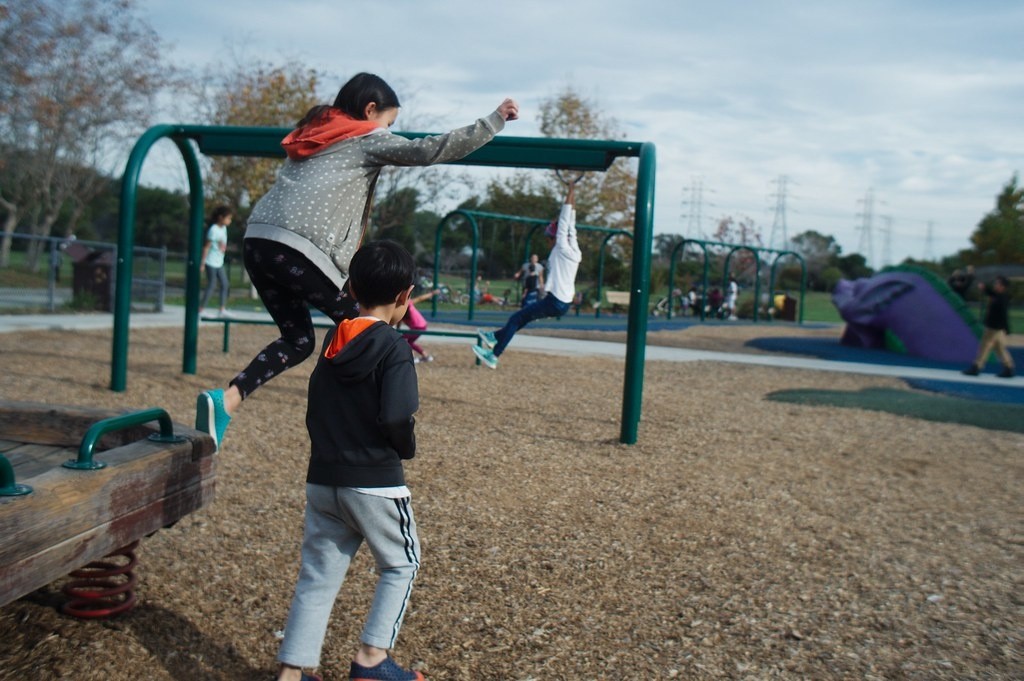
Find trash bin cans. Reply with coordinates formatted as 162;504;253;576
56;234;114;313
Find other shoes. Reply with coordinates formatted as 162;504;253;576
996;367;1016;378
421;355;434;362
962;364;980;375
413;357;419;363
349;654;425;681
300;671;323;681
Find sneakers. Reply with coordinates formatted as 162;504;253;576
194;387;232;456
471;344;498;369
477;328;498;348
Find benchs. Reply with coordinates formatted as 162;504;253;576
606;290;630;312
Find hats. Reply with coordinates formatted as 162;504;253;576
543;220;558;237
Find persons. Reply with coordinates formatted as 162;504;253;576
472;178;582;369
653;276;777;322
965;276;1016;378
198;206;233;316
416;253;544;305
949;264;976;302
275;240;427;681
397;289;440;363
197;73;519;456
